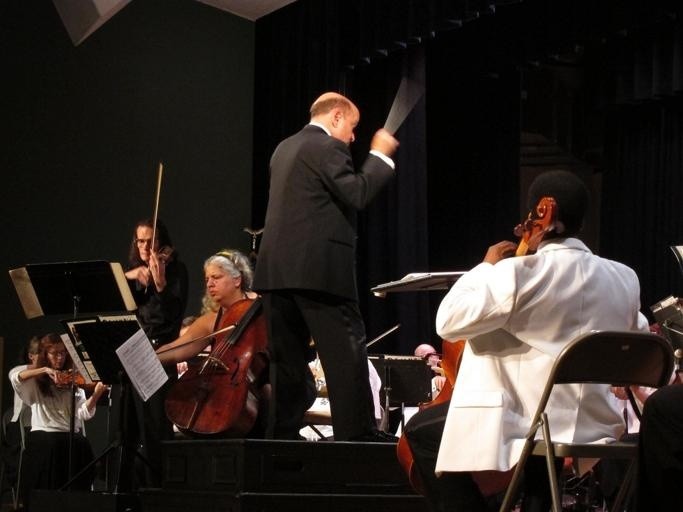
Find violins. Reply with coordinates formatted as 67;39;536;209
154;247;176;264
55;372;112;390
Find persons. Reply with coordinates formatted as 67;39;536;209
116;222;189;495
246;90;400;439
8;312;446;512
405;165;641;510
121;247;267;491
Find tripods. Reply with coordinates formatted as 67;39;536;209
58;383;165;491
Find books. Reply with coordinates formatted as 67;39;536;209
5;257;137;322
372;264;470;295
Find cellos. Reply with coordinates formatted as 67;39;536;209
164;296;269;434
396;196;558;497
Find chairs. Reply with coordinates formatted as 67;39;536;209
490;330;674;510
9;400;97;510
